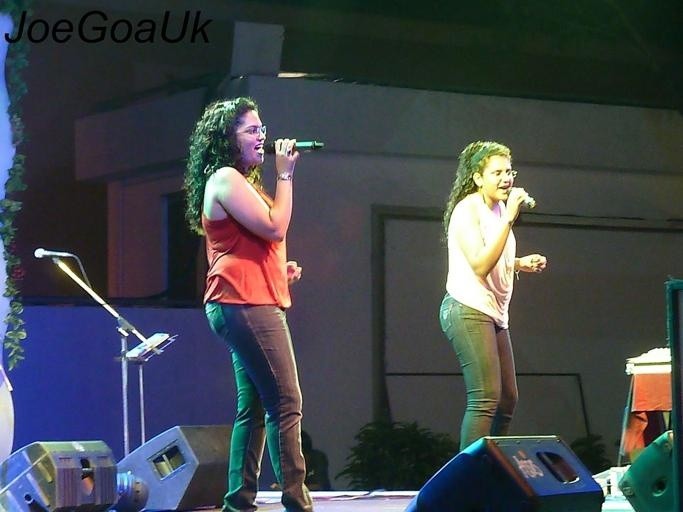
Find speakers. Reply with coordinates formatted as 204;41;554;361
617;431;675;511
115;425;234;512
0;441;116;512
402;434;604;512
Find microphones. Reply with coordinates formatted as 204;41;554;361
507;187;536;209
35;248;69;258
263;140;325;154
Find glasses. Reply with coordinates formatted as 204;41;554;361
235;125;266;137
481;169;517;178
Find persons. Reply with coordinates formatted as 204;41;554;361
435;140;548;454
185;95;315;511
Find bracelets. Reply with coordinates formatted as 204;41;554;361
275;171;294;181
513;258;520;282
